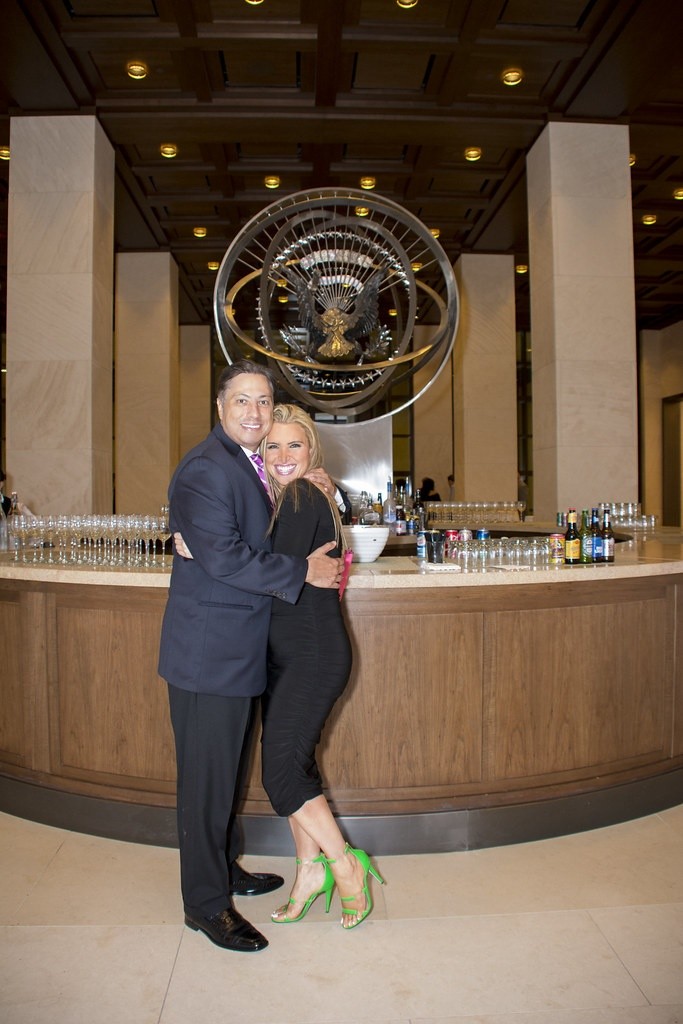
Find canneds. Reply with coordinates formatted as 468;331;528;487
407;518;416;534
549;533;566;564
416;528;490;560
557;512;568;527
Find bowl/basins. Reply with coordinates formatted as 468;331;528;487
342;524;389;563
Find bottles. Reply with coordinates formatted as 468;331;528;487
6;492;21;518
357;476;425;536
551;533;565;564
0;492;7;551
590;508;602;563
579;508;592;563
416;526;490;566
601;508;615;562
565;507;581;564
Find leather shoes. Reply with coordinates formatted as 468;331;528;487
229;865;284;897
185;906;269;952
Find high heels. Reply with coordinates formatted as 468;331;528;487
271;853;335;923
326;841;384;929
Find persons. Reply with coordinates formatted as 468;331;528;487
157;359;343;952
395;476;455;521
173;403;383;931
0;471;42;550
518;471;527;520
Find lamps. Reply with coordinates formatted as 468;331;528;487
126;0;683;324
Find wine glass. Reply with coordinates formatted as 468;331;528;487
425;501;526;523
0;504;171;569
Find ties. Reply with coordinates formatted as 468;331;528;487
250;454;272;500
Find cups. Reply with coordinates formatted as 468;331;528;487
598;502;656;528
452;537;560;568
